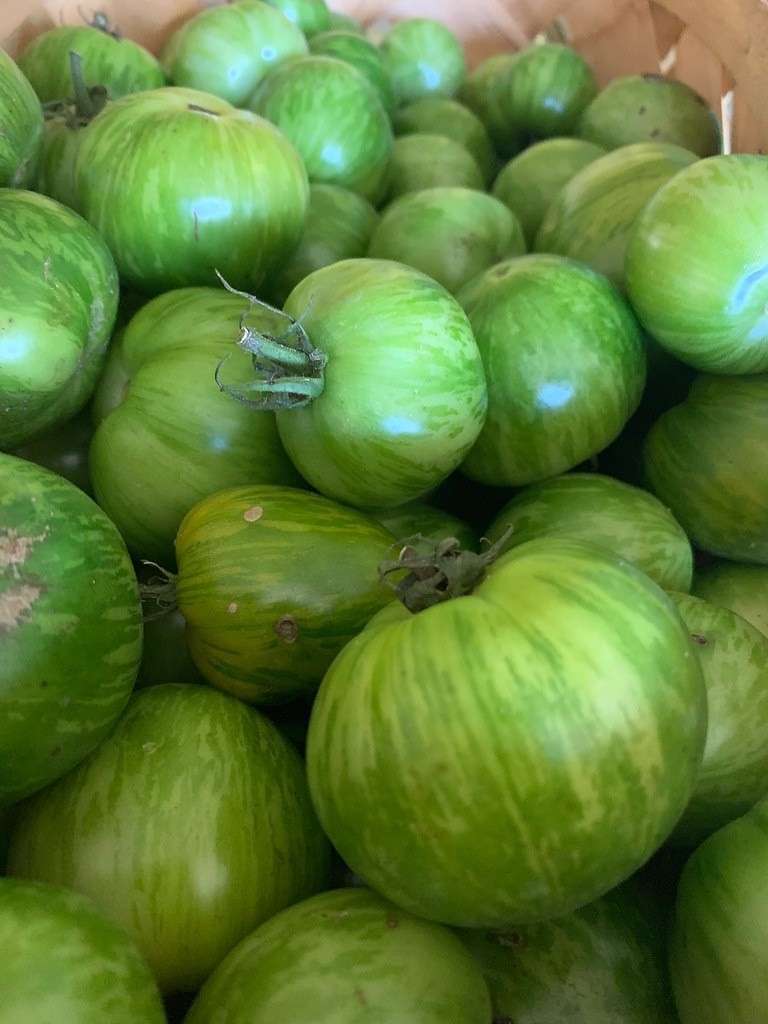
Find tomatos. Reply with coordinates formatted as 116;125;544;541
0;1;768;1024
